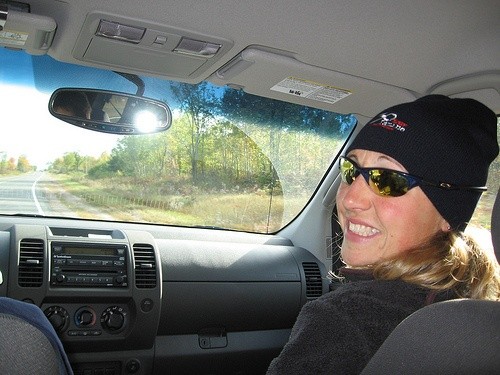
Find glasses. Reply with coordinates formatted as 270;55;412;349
338;155;488;198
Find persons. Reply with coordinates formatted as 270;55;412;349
263;93;500;375
52;89;91;120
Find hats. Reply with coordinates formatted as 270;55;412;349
346;94;500;232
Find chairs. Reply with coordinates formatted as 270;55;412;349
352;182;500;374
